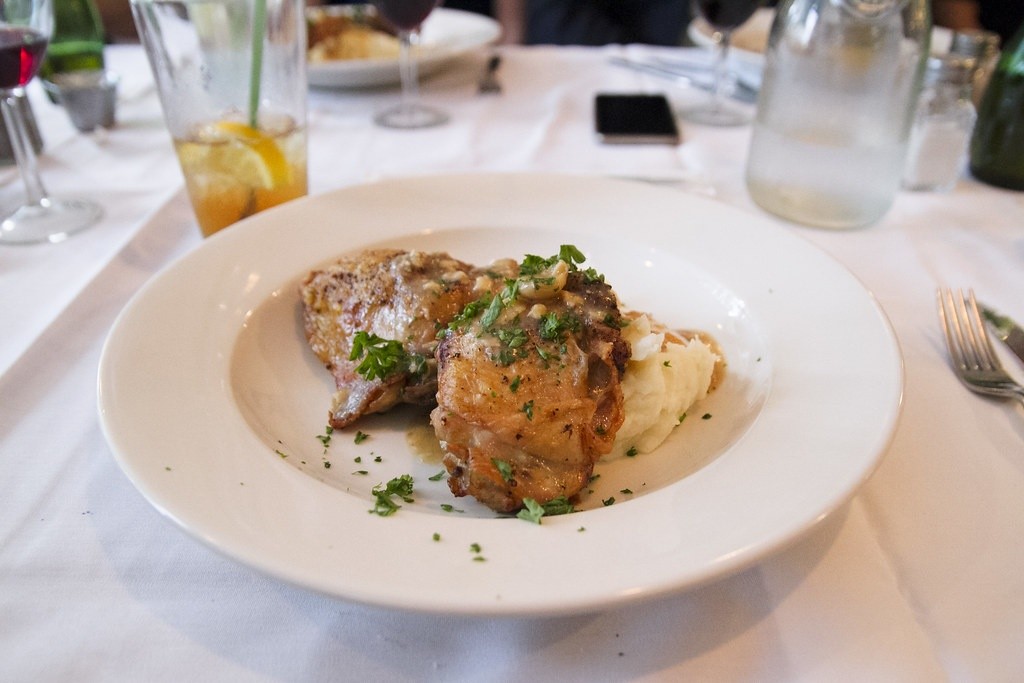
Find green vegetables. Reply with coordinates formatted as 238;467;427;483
275;243;711;563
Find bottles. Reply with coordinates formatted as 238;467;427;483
903;23;1024;191
743;0;931;227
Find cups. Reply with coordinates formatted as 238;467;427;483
129;1;310;237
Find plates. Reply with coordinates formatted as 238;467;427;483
95;168;908;617
304;5;505;89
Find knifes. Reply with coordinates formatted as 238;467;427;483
964;297;1024;362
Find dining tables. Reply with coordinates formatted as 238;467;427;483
0;46;1024;683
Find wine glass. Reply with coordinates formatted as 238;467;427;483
0;0;108;246
367;0;452;128
677;0;761;126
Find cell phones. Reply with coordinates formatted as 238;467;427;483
591;90;681;147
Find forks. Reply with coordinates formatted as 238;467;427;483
935;286;1024;408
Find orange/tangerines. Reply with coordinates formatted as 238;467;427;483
178;122;309;237
